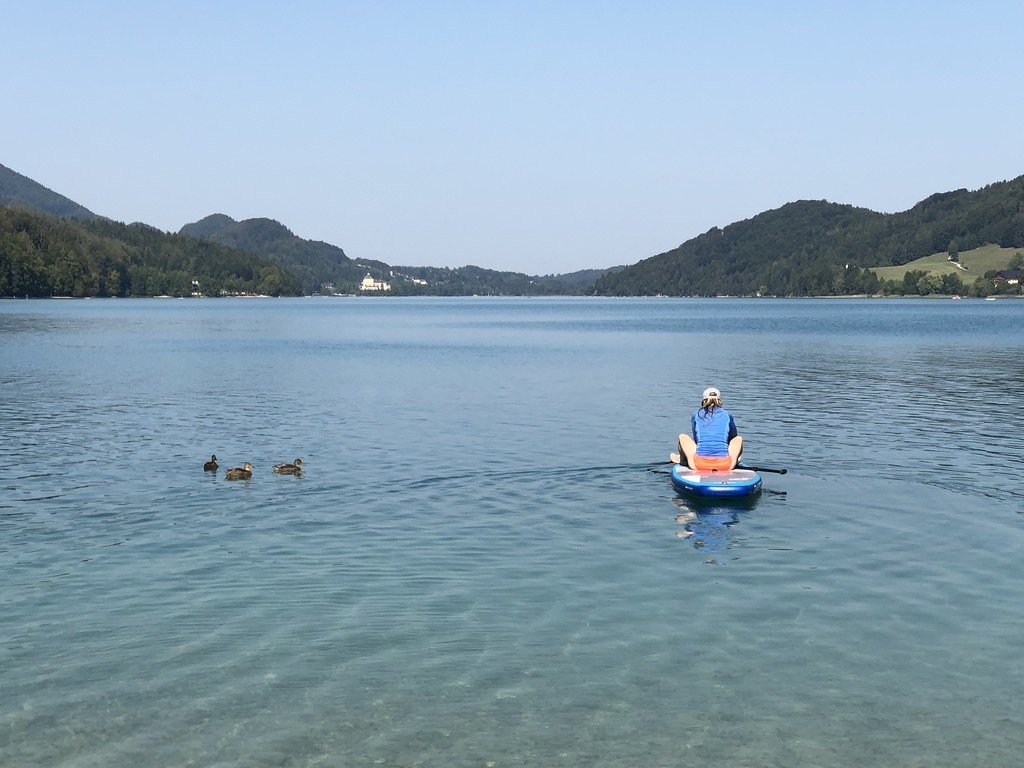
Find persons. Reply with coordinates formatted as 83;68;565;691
669;387;742;472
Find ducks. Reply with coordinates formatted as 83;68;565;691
272;458;303;474
203;454;218;471
225;462;256;480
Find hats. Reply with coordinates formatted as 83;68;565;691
703;387;720;399
